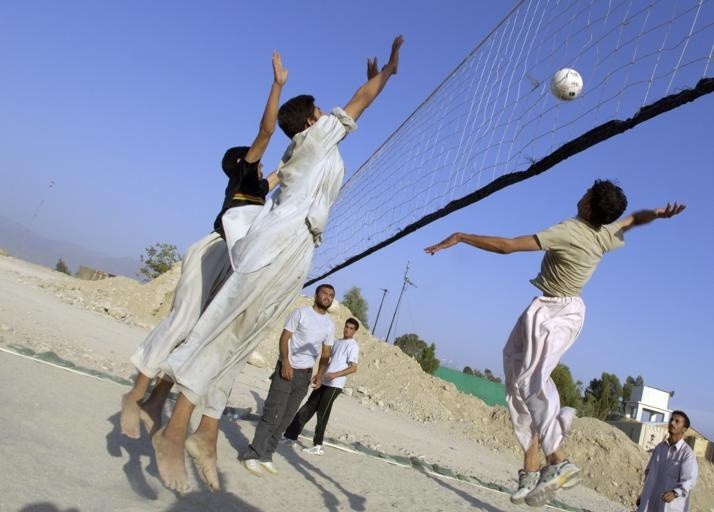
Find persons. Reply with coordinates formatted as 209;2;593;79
118;48;289;441
240;284;336;477
150;34;404;495
633;410;698;512
276;317;360;457
423;177;686;505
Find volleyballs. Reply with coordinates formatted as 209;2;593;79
550;68;585;102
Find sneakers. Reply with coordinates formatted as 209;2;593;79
525;459;583;507
260;461;278;474
245;458;263;478
510;468;542;505
278;438;297;448
301;445;324;456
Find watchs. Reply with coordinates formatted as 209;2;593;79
672;490;678;498
